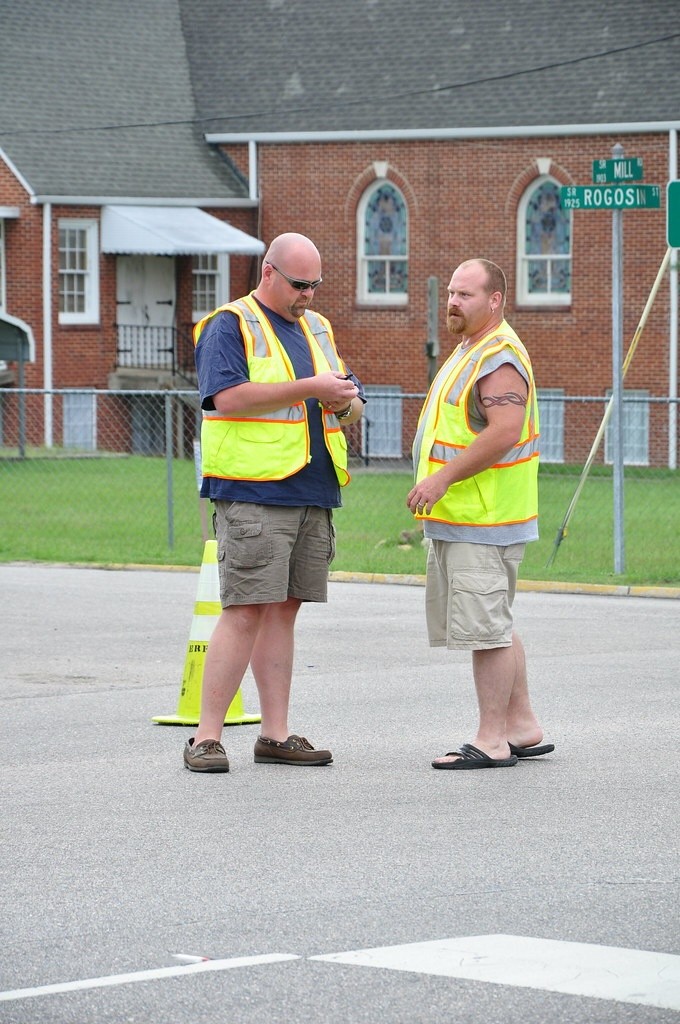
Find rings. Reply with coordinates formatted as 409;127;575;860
418;503;423;507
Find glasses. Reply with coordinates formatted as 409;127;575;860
266;261;322;291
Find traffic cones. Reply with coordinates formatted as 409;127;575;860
149;538;263;727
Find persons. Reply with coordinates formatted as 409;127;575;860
406;258;555;769
183;232;368;771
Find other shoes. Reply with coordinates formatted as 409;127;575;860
254;735;333;766
183;738;229;772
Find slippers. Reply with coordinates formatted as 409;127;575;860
432;743;518;770
507;740;555;757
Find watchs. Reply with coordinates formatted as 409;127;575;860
336;403;352;419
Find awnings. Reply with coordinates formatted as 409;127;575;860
99;206;265;256
